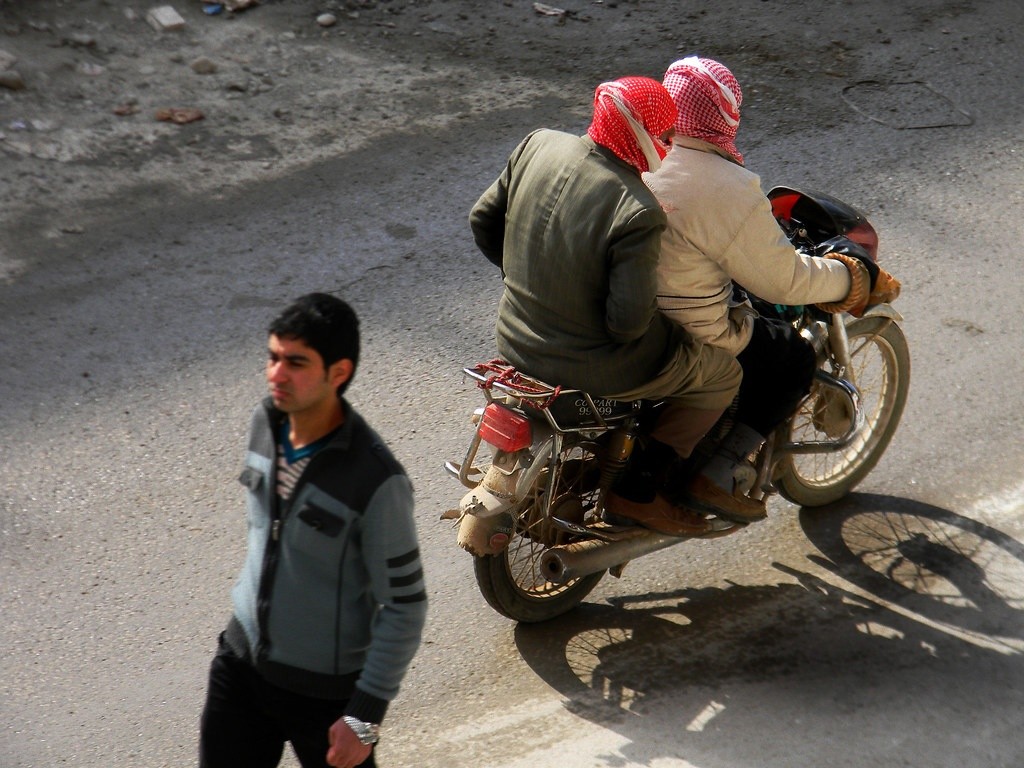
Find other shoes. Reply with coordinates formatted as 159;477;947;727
602;493;713;538
684;471;768;524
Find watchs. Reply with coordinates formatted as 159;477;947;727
341;715;381;747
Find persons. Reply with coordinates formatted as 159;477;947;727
647;52;904;525
470;76;745;539
187;291;430;768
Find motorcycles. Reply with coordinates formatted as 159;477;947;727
439;185;911;624
